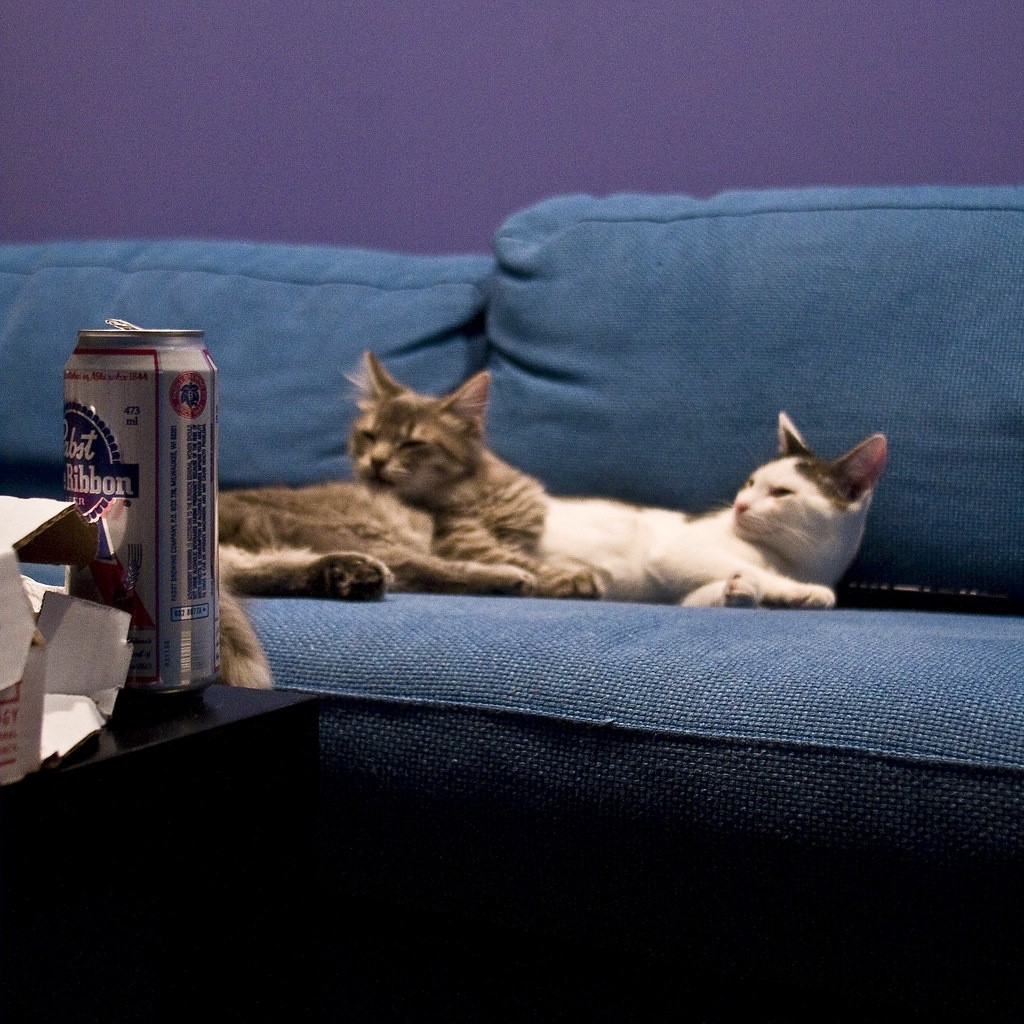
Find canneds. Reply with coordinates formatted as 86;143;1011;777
61;318;222;697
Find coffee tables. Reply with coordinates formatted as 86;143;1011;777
0;682;321;1023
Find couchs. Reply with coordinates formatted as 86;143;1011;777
0;189;1024;1024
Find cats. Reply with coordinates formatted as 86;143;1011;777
215;349;603;692
541;409;885;608
